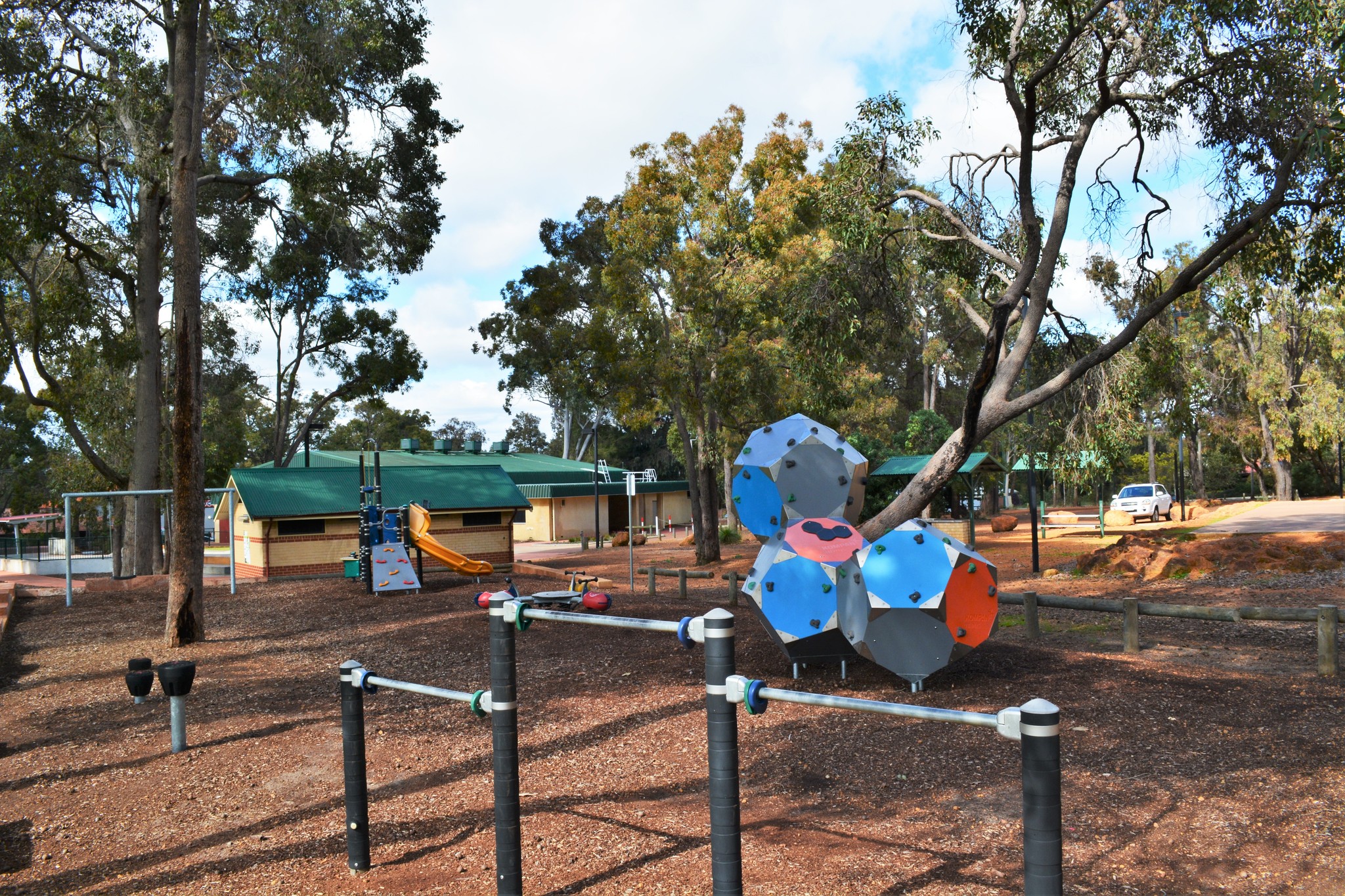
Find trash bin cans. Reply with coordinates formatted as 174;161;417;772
48;538;76;555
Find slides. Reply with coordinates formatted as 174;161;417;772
410;528;493;584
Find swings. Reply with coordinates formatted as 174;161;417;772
107;497;138;581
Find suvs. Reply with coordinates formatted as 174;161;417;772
1110;481;1173;523
